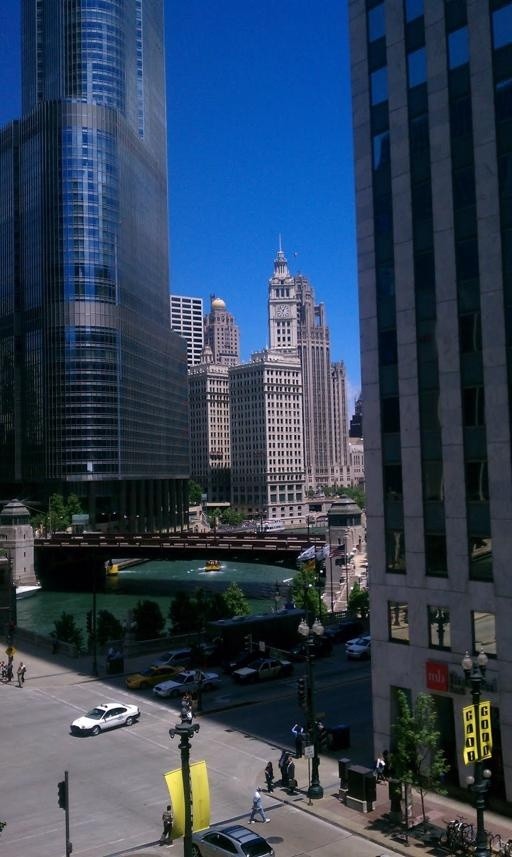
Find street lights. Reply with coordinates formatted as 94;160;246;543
297;616;325;799
460;647;494;857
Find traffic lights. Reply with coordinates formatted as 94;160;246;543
58;781;66;808
296;677;307;702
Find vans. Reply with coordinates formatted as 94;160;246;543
155;643;209;667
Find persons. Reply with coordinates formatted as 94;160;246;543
0;660;26;688
278;750;299;795
206;559;221;565
242;520;257;528
374;752;387;786
313;721;324;753
248;786;271;824
291;724;303;759
263;761;274;794
159;805;174;845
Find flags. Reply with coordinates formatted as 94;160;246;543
315;543;330;562
297;545;315;561
328;544;345;559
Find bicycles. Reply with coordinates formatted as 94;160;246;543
439;814;512;857
0;665;13;682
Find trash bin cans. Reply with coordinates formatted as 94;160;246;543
106;657;124;675
329;725;352;751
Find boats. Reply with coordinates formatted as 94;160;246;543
204;558;221;571
256;520;286;533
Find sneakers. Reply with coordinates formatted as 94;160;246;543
248;820;255;823
264;819;271;823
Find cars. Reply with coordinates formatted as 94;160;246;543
191;823;274;857
153;668;219;697
232;657;292;683
71;703;140;736
126;664;185;690
345;635;371;659
